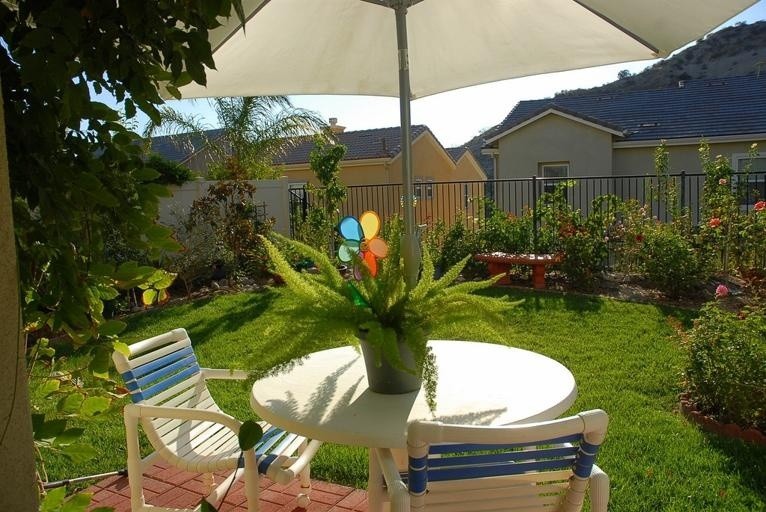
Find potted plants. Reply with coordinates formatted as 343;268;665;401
258;223;529;416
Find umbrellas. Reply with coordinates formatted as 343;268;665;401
109;1;760;292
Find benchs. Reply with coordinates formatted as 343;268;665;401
472;251;566;289
